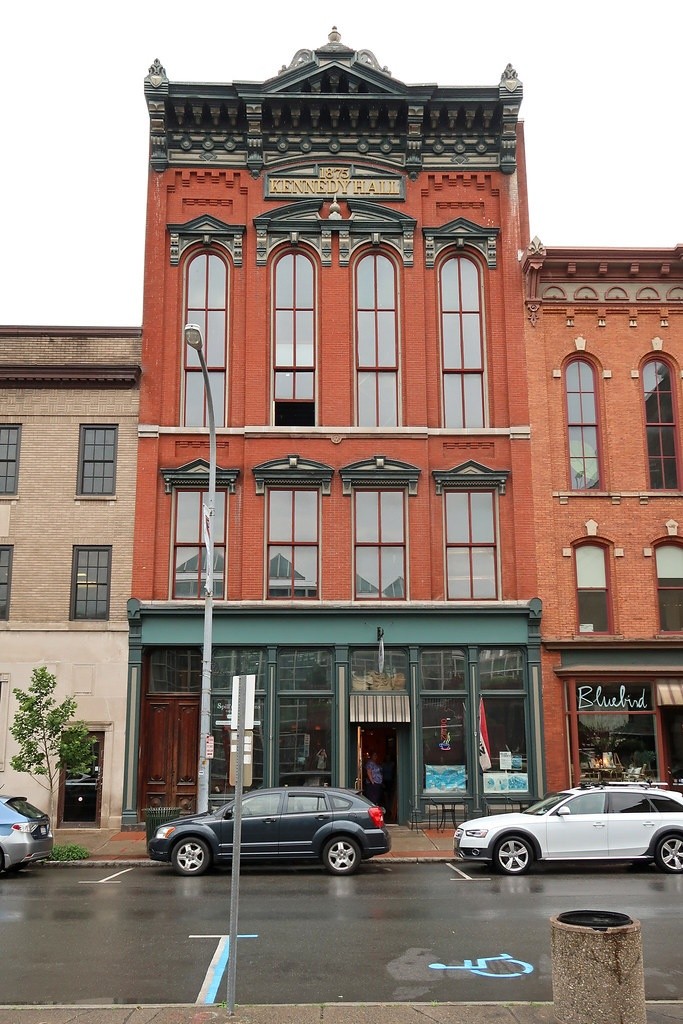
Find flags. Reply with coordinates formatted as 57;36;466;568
477;698;492;772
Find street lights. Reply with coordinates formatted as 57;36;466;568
186;324;217;814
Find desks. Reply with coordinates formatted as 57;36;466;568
501;792;542;813
430;798;464;831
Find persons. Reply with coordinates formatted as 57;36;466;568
366;752;384;805
382;755;395;817
316;748;328;770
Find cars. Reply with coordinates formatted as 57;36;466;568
452;781;683;874
0;794;54;876
148;785;391;876
248;770;332;786
65;769;98;807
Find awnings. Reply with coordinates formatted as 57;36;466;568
655;678;683;707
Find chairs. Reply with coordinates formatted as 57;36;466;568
462;793;488;822
408;794;438;834
